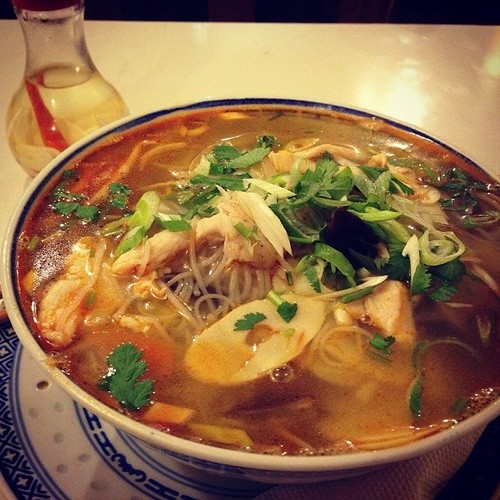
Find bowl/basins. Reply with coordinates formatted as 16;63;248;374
2;95;500;487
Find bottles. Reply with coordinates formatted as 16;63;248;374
7;1;131;182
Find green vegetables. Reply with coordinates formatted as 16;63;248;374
50;132;470;421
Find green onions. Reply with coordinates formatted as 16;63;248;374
461;209;498;228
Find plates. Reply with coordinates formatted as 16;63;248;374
0;301;500;500
